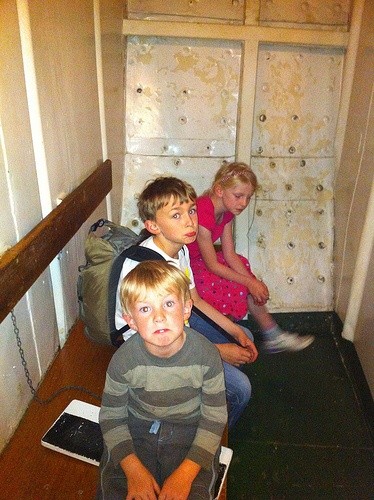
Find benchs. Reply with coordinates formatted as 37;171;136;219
0;319;230;500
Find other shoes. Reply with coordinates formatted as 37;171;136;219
261;330;314;354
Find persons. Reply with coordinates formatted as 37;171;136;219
98;260;228;500
115;175;259;431
186;160;315;354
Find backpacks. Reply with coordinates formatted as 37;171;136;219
75;218;167;348
